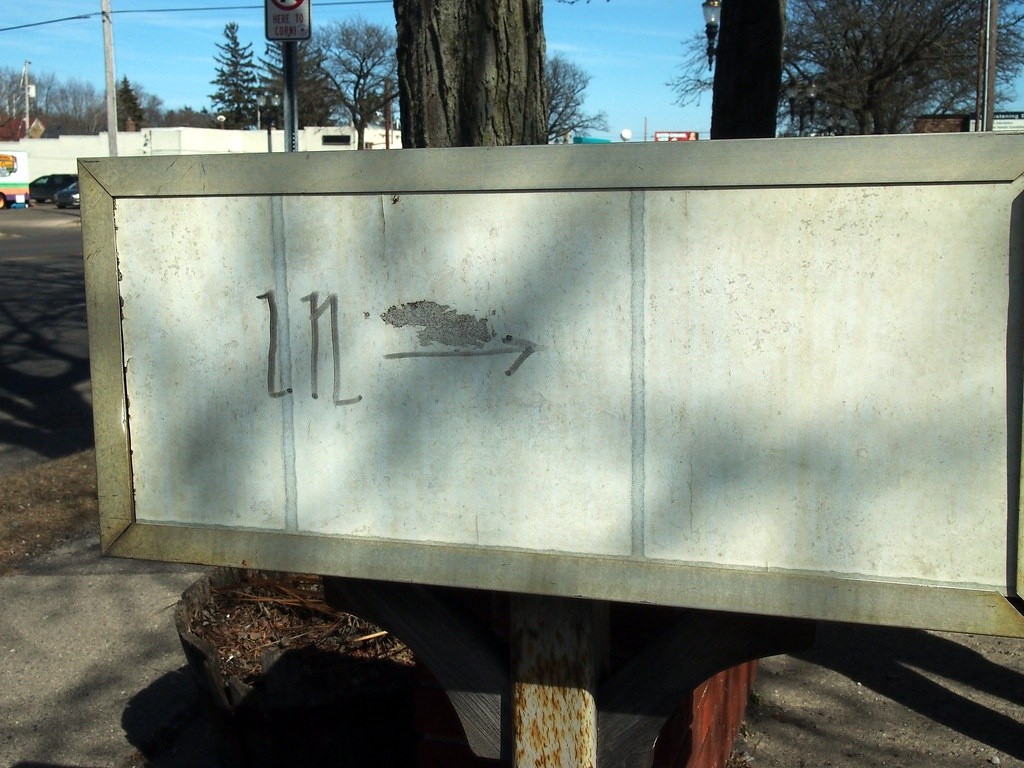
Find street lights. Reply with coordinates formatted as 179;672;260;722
256;91;281;153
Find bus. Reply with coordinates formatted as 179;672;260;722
0;150;30;209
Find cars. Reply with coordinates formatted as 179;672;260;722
56;182;79;209
29;174;78;203
